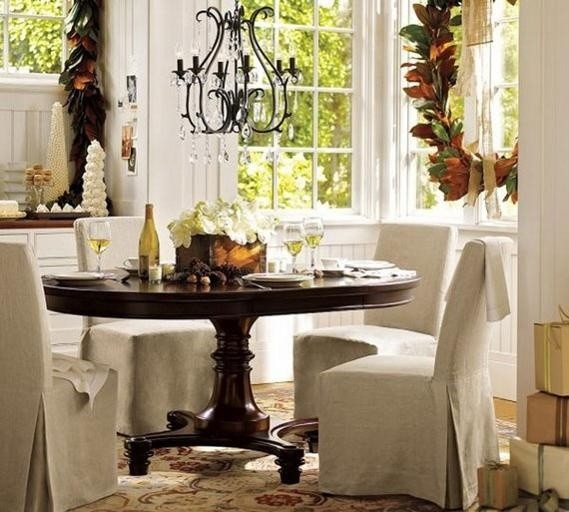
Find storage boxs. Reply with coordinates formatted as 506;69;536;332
508;436;569;504
526;391;569;447
534;321;569;398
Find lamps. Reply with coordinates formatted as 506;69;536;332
170;0;303;165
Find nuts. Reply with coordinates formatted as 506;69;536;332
313;269;323;278
200;277;210;285
187;275;197;283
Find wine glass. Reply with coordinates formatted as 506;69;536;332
285;224;306;273
305;220;324;272
88;220;112;280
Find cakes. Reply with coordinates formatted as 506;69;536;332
0;201;19;217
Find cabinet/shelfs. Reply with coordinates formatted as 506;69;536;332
0;228;84;344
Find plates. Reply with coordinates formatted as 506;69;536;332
241;272;316;289
346;260;395;270
45;272;113;288
316;267;348;278
113;265;138;276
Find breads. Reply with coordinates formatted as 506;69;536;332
24;165;52;186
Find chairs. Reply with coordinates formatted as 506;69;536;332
74;217;219;435
315;236;515;509
0;242;117;512
292;224;457;420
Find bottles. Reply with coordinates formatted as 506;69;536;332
138;203;160;280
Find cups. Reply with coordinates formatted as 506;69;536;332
122;257;139;269
320;257;342;270
